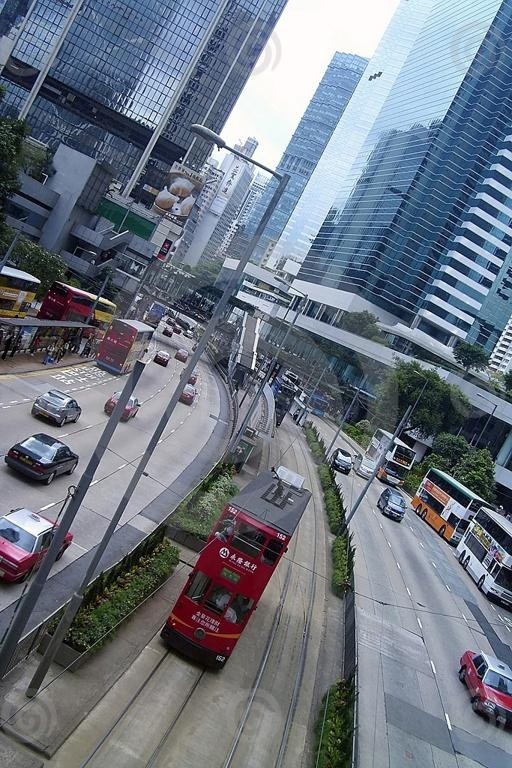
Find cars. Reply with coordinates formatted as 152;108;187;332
458;650;512;728
5;433;79;485
32;389;81;427
377;488;407;522
104;391;140;422
330;448;375;480
153;318;199;405
0;507;73;584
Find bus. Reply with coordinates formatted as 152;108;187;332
0;265;166;375
160;466;312;674
256;353;329;425
411;467;512;611
365;428;416;487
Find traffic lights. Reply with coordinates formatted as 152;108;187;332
156;239;172;262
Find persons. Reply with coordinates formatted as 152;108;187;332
213;587;233;612
0;323;102;365
238;596;250;616
213;525;234;543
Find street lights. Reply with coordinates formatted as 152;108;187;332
457;393;498;481
229;275;308;454
28;123;291;704
398;371;428;443
